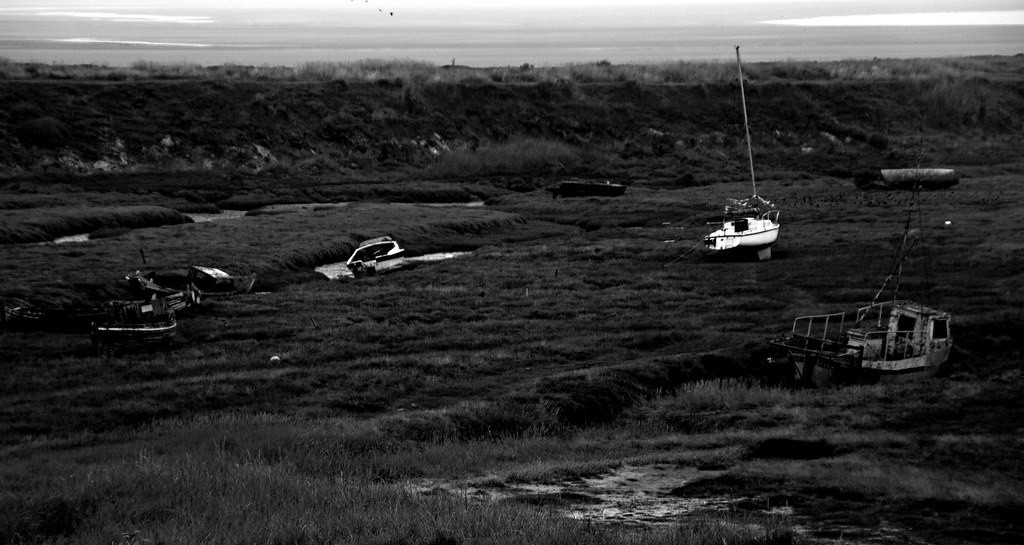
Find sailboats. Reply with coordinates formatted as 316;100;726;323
771;165;955;372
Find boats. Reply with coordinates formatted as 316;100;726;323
560;179;627;197
704;45;778;259
93;301;176;345
134;266;256;298
347;240;405;275
